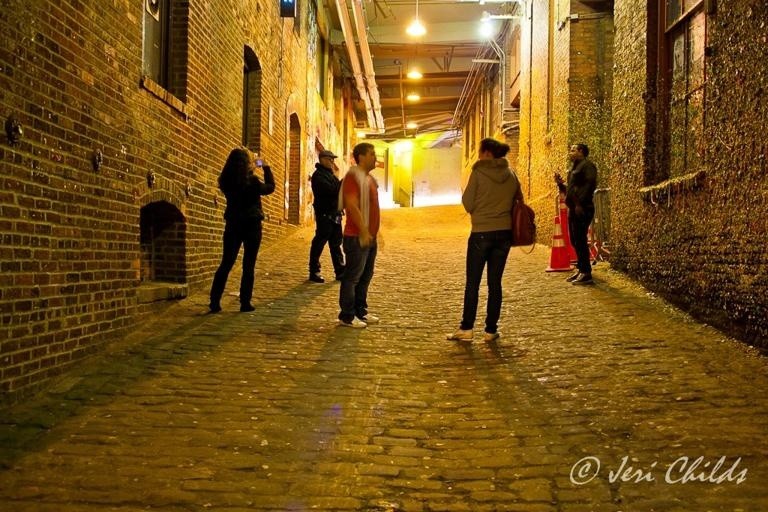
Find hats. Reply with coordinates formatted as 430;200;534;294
318;150;338;158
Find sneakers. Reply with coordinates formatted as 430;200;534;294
339;315;367;328
566;271;579;282
446;329;473;343
361;314;379;323
572;273;593;285
485;332;499;341
208;302;222;311
240;304;256;312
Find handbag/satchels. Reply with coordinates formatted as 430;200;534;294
511;202;536;245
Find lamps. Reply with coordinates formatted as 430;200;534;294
407;112;417;128
406;61;422;82
405;0;427;36
406;88;420;101
478;10;523;40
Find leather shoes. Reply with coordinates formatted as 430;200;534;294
309;273;326;283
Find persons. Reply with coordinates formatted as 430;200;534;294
209;148;275;313
446;138;520;343
307;150;344;283
553;143;597;285
338;143;380;328
